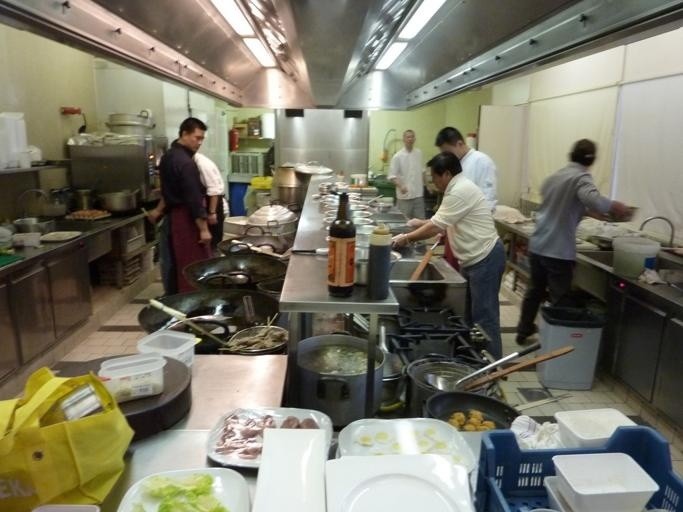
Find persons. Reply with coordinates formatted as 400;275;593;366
390;151;505;362
158;117;211;294
386;129;430;220
433;126;496;321
146;139;224;249
514;139;631;347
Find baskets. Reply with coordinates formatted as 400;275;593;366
475;425;683;512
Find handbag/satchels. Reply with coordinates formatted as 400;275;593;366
0;366;135;510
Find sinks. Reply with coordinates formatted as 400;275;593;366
577;251;683;270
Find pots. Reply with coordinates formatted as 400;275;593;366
241;203;298;240
102;109;158;135
97;192;136;211
216;225;293;259
137;280;278;352
298;315;527;429
266;160;308;211
182;243;286;291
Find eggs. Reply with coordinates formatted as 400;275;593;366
73;210;108;220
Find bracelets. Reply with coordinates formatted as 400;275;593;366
208;210;217;215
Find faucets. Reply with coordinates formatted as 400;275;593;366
639;216;674;245
16;189;48;202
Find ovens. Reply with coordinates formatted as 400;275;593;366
67;134;168;203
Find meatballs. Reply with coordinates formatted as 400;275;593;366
445;408;496;432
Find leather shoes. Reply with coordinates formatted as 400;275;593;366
517;322;536;345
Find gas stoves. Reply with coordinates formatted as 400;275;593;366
348;307;495;395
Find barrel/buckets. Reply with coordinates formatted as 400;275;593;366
612;235;661;278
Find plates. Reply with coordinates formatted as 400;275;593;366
40;231;82;243
206;407;333;471
304;416;480;510
115;466;250;511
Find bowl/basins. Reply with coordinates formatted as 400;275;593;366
541;451;655;510
12;217;55;233
553;405;637;447
552;432;565;449
319;181;399;286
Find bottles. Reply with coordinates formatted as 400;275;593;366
285;60;326;299
466;134;477;149
367;218;391;301
62;106;86;158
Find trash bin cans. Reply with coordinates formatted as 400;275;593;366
227;172;261;216
537;308;604;391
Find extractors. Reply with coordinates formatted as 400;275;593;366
0;1;683;114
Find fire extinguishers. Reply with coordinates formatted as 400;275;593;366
229;125;239;151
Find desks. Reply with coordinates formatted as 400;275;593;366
91;423;513;512
